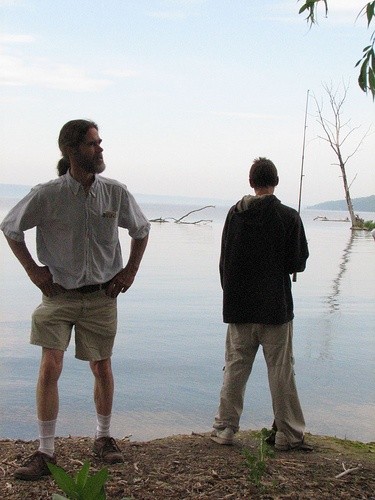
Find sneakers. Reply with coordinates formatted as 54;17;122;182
93;435;124;464
13;450;56;481
209;427;235;445
274;431;289;451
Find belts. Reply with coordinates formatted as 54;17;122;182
74;278;112;294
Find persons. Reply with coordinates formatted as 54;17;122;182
209;157;309;450
0;119;150;481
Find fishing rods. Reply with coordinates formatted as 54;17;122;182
292;90;311;283
